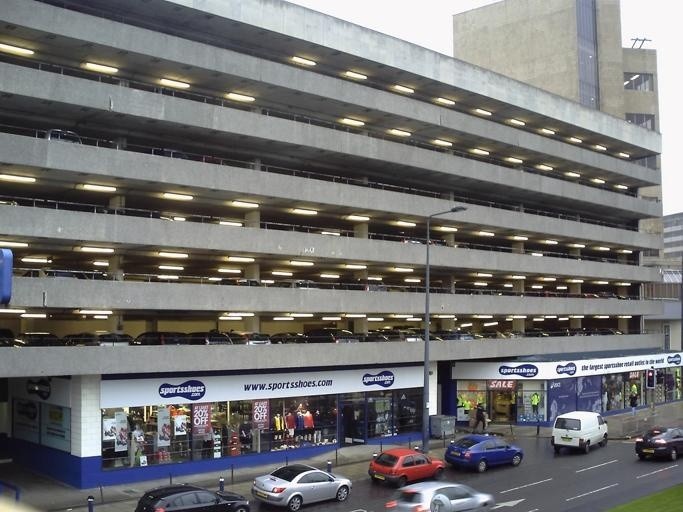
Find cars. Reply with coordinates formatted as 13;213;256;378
21;265;111;278
386;482;494;512
252;463;352;512
445;434;523;472
1;326;624;347
222;276;629;301
635;426;683;461
368;448;446;486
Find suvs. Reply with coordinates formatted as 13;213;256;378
134;482;250;512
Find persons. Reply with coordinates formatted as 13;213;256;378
530;393;540;415
473;399;487;435
627;391;639;417
269;408;322;446
461;399;472;415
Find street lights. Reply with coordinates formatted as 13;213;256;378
421;206;468;450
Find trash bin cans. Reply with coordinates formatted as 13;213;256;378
431;415;457;440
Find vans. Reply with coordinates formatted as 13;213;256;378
551;410;608;454
